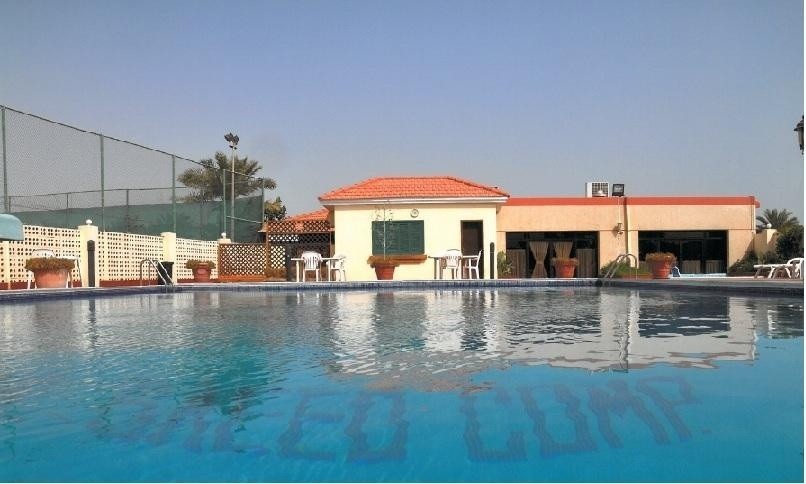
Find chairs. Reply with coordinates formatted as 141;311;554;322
301;250;346;282
439;248;483;280
753;257;804;279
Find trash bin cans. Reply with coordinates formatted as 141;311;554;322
157;262;173;285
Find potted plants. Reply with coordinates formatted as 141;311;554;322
366;254;399;280
646;251;677;279
186;258;216;283
26;256;75;288
551;256;580;279
